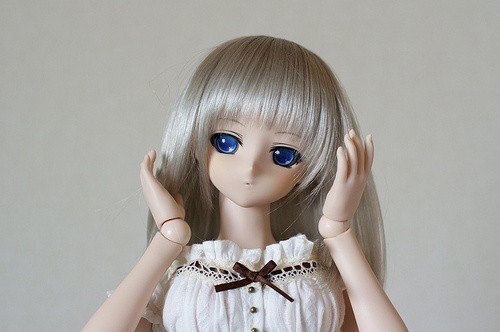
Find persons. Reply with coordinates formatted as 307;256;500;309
78;31;415;332
